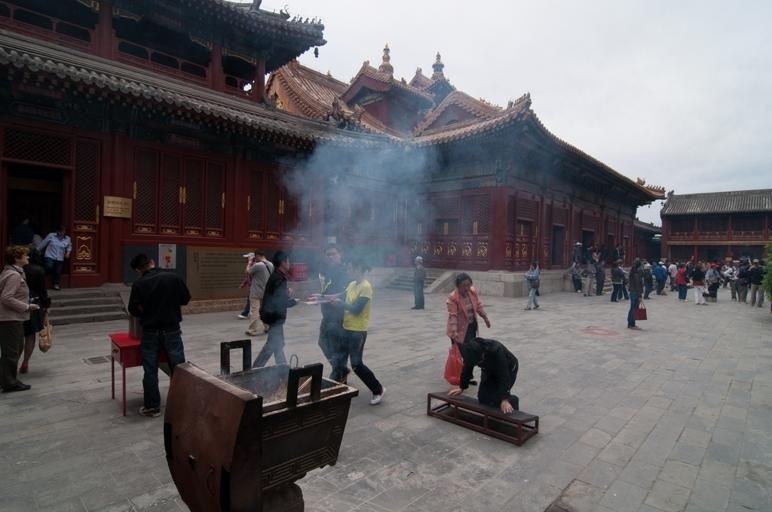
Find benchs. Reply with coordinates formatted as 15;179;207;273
427;388;541;447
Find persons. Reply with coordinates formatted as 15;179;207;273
445;273;492;387
0;222;72;394
126;250;190;416
410;255;427;310
523;242;771;329
237;243;387;405
454;334;523;413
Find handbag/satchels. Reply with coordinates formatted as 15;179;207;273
39;326;52;353
636;308;647;320
530;279;539;287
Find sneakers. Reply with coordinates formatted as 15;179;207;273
370;388;386;405
4;382;30;390
238;315;249;319
138;407;160;418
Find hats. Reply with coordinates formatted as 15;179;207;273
243;253;255;258
416;256;423;265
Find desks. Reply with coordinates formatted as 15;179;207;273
109;334;169;418
